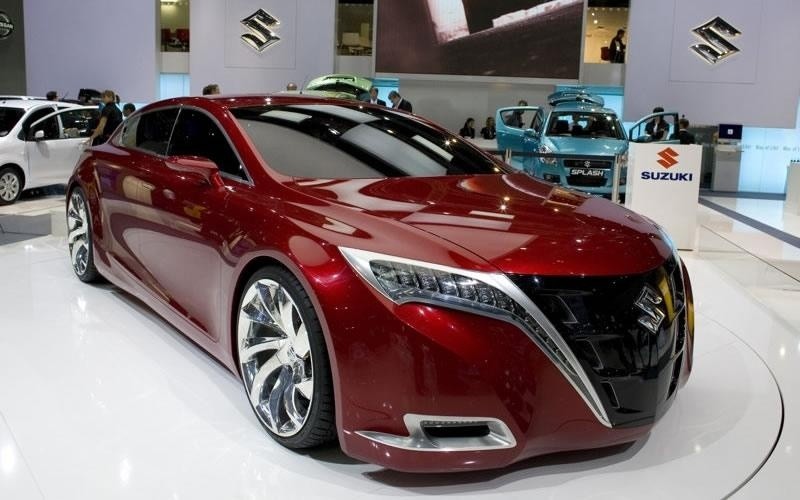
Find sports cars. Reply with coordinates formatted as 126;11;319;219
66;94;694;474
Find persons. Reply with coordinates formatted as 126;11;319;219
503;100;528;128
669;117;695;143
47;90;59;101
79;89;123;145
123;103;135;117
646;107;669;141
389;90;412;113
480;117;496;138
203;83;220;94
366;86;386;106
459;118;475;139
611;29;626;63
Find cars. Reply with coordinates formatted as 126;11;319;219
495;87;680;200
1;95;99;204
277;74;371;103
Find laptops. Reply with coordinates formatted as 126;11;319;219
718;124;742;139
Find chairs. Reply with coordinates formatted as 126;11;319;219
549;119;611;138
